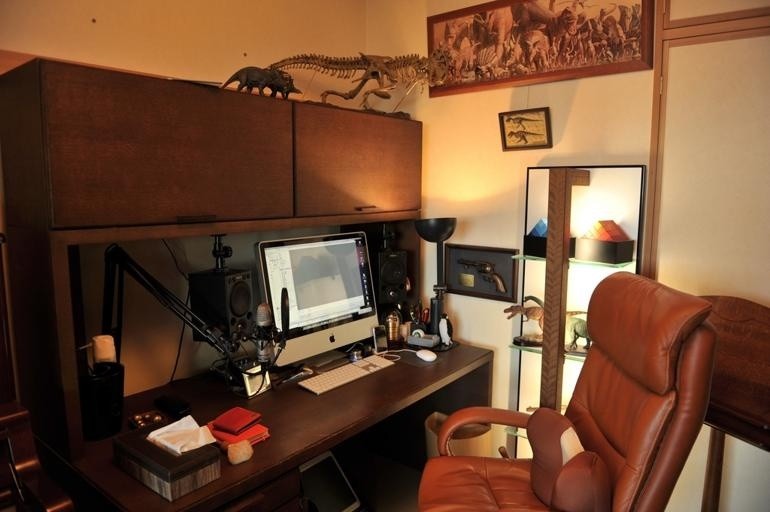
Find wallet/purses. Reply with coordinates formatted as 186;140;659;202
212;407;263;435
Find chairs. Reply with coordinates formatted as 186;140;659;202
418;274;714;511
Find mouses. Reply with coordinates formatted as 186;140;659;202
415;348;439;364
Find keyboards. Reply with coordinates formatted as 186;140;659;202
295;355;396;397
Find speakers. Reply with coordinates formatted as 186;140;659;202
373;247;417;318
186;268;257;345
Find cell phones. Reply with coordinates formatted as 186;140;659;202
375;325;389;355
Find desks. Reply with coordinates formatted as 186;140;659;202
63;322;492;511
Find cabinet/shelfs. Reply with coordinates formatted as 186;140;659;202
2;58;294;228
294;101;423;217
503;167;647;456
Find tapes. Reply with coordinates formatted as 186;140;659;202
412;329;425;337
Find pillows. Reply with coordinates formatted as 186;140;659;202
525;407;613;511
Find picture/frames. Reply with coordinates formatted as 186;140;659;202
444;242;519;304
498;108;551;151
427;0;653;97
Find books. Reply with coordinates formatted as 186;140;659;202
206;417;270;454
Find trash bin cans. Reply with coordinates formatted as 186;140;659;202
424;411;492;458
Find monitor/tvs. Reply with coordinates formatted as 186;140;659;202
298;451;363;512
253;229;379;369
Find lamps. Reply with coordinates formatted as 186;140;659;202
414;219;458;354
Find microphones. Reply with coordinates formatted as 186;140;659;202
254;303;275;364
